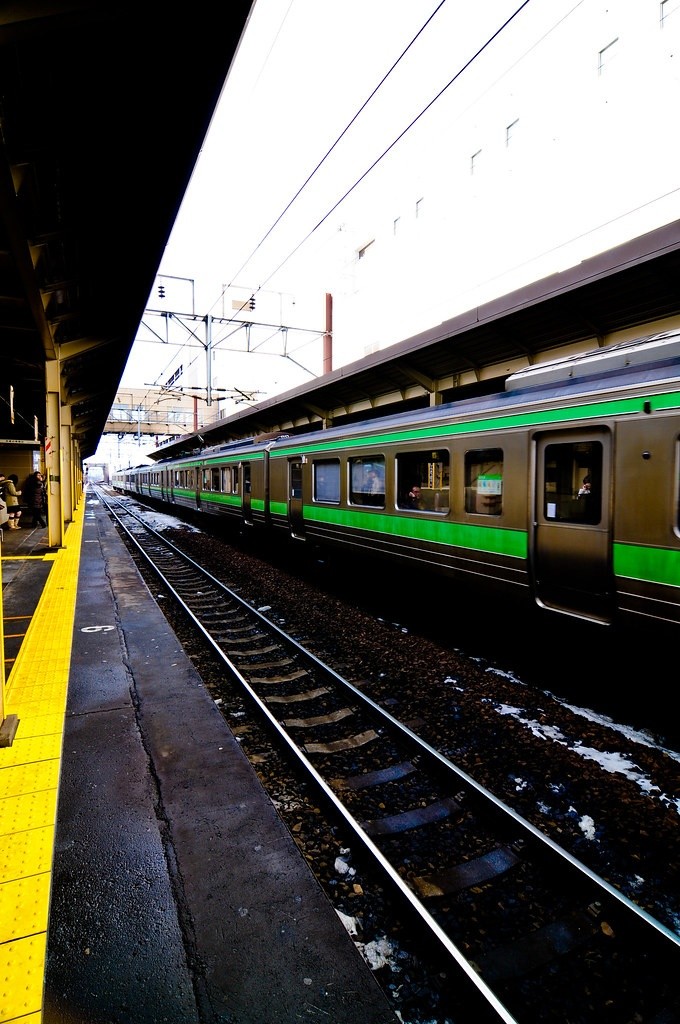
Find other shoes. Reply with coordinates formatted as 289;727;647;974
32;524;47;528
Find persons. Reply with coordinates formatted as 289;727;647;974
578;471;591;496
0;471;48;529
404;484;430;510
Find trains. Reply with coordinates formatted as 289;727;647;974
111;329;680;627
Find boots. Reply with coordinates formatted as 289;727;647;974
8;519;17;529
14;519;21;529
